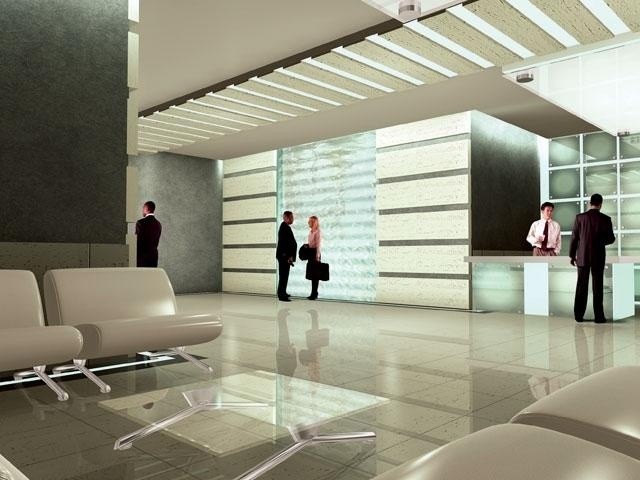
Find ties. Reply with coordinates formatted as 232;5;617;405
542;221;548;250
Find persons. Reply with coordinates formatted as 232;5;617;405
136;201;162;267
304;215;322;301
568;193;616;323
525;202;562;256
275;210;298;302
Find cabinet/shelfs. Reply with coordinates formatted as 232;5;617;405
546;132;640;302
465;255;640;322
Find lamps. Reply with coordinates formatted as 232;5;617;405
517;69;534;83
618;128;630;136
399;0;421;19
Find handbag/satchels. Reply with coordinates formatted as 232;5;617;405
306;261;329;281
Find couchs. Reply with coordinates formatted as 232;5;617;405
42;267;223;393
0;268;83;401
510;364;639;459
371;424;639;480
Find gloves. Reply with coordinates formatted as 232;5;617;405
570;258;576;267
